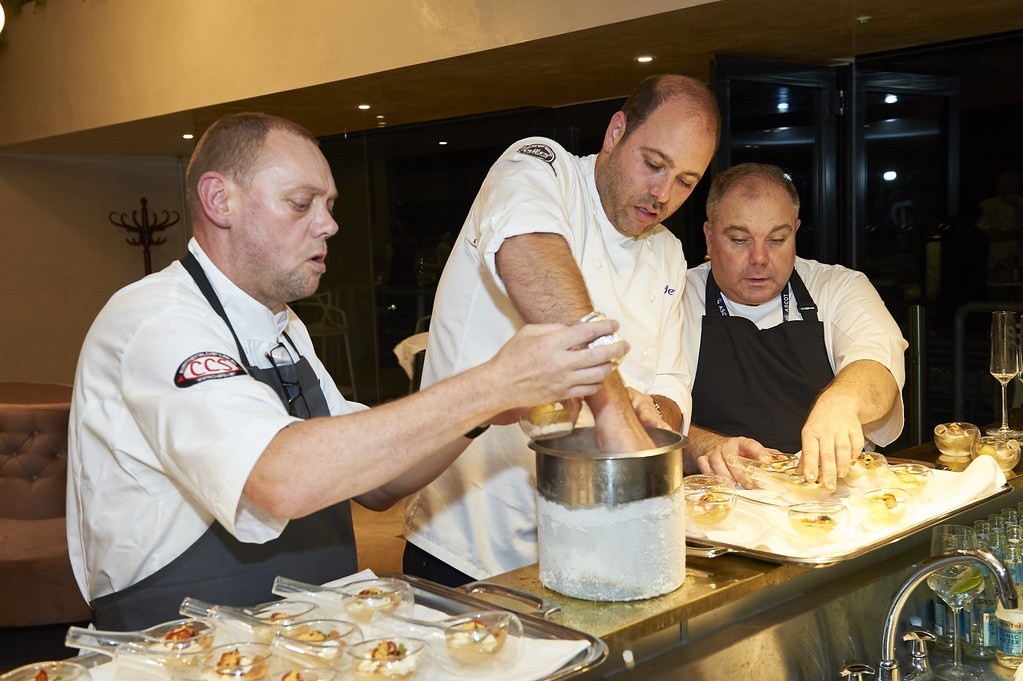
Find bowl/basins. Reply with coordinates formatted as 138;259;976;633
843;453;887;487
970;437;1021;473
515;397;582;440
788;503;850;541
887;463;935;494
934;422;981;456
862;488;911;524
683;474;737;525
528;425;690;603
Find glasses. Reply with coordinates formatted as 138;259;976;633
265;341;310;420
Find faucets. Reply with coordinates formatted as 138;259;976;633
875;548;1018;681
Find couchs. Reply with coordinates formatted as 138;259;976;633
0;404;94;630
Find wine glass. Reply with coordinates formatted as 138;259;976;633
986;311;1019;436
927;525;984;681
1014;316;1023;446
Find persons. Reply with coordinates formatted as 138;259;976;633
434;229;458;281
687;163;909;492
385;220;418;334
403;74;719;591
974;173;1023;271
66;112;630;657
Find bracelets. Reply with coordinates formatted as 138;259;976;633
650;396;665;420
464;424;491;439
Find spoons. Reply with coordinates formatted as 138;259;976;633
112;642;271;681
371;609;524;678
179;596;325;629
0;661;94;681
727;452;800;489
746;466;831;503
64;618;218;657
206;604;365;681
269;632;437;681
271;576;414;640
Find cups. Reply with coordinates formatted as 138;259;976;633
567;311;629;373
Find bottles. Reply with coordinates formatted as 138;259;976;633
932;502;1023;670
